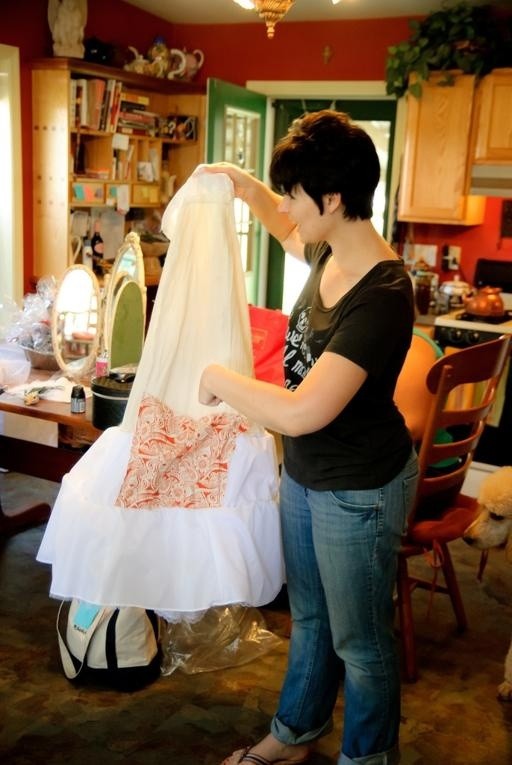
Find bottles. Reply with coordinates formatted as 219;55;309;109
71;385;86;414
82;240;93;271
91;222;105;277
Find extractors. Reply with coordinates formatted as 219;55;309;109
467;164;512;197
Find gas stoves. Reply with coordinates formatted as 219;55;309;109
431;293;512;335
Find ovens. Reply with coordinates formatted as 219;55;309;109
434;325;512;468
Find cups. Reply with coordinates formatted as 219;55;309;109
415;269;439;316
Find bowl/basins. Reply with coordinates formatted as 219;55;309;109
19;338;61;373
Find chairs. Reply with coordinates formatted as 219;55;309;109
394;333;510;685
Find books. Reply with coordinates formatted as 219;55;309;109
69;75;159;180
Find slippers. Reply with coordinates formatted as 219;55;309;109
221;738;311;764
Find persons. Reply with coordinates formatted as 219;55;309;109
194;107;421;765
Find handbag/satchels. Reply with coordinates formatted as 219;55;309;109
249;306;289;387
56;598;163;691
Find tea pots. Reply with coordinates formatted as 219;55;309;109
460;284;506;318
121;34;205;83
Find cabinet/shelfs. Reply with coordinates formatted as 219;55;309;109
469;68;512;164
394;68;483;227
29;58;213;292
